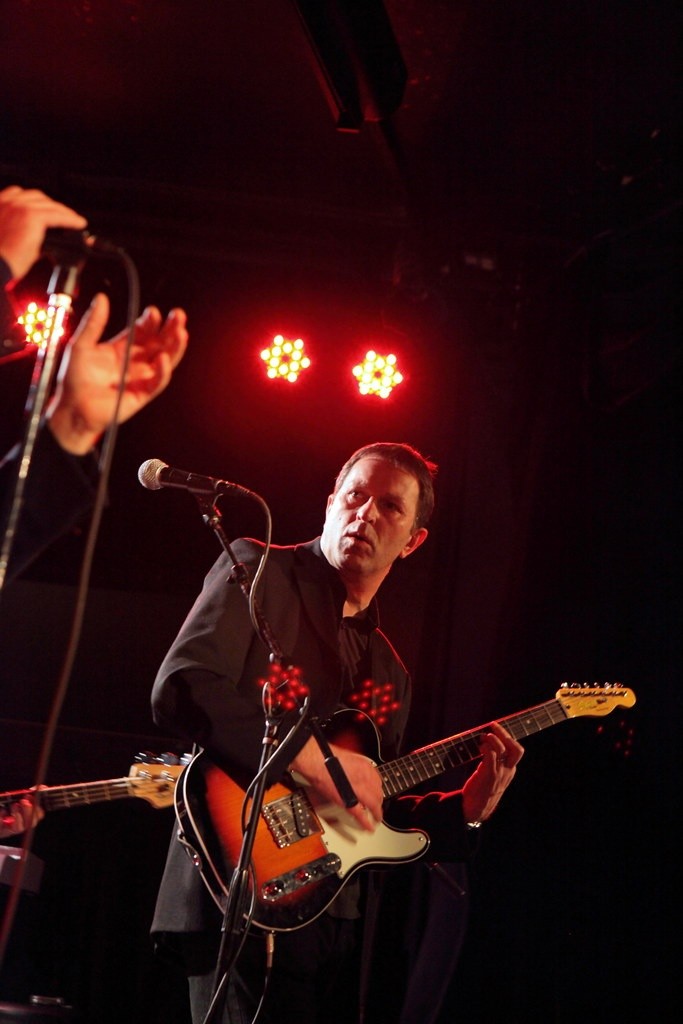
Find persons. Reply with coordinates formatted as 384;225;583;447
0;184;189;592
151;441;527;1024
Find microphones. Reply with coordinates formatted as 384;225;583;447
138;458;253;500
43;225;117;263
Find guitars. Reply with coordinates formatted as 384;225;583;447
172;679;639;933
1;749;189;843
